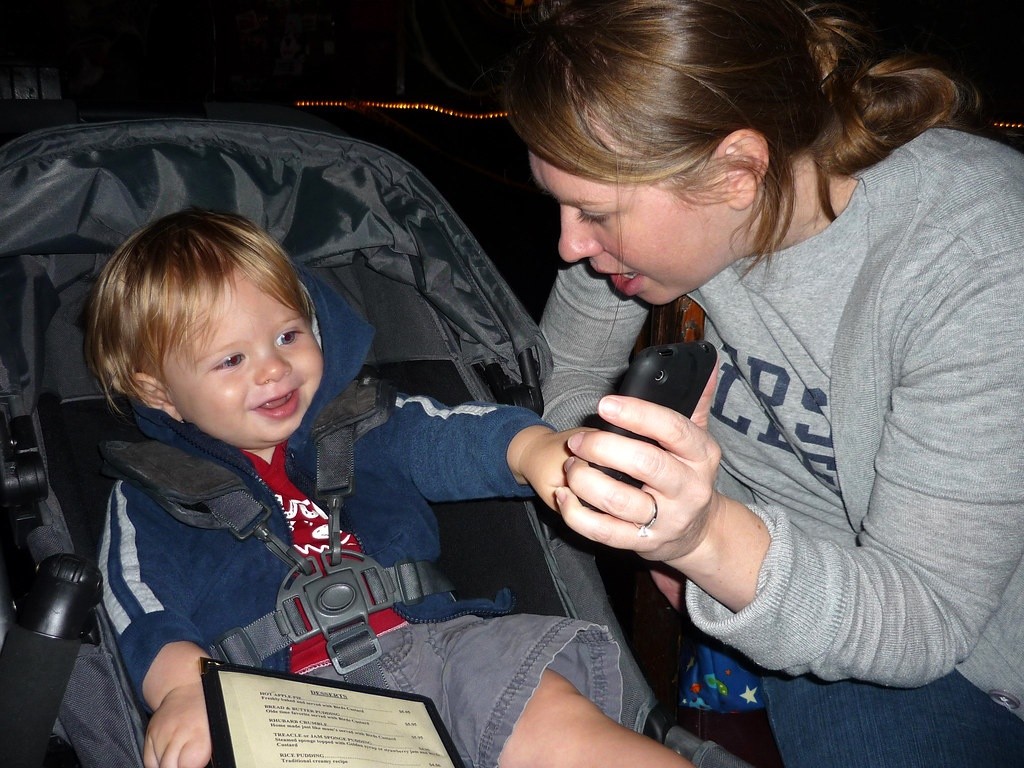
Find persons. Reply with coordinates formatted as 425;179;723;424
82;206;698;768
501;0;1024;768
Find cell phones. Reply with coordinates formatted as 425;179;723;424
575;339;718;510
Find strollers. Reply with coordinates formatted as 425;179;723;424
0;115;758;768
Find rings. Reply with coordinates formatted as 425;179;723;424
634;492;657;537
